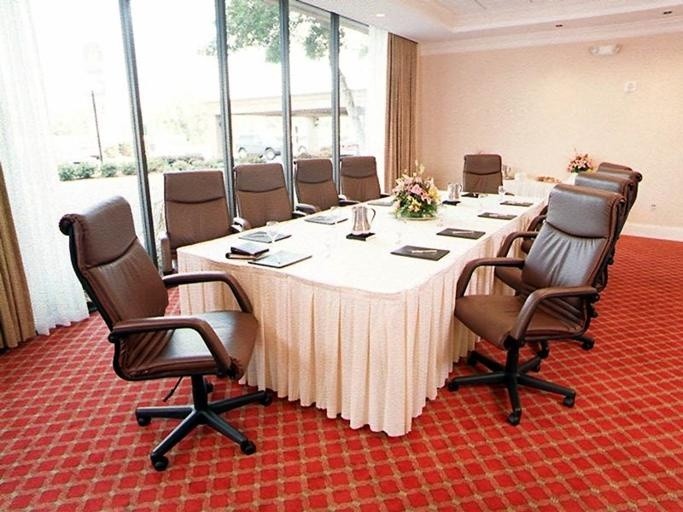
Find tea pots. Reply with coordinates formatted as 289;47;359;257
351;204;377;235
447;183;463;202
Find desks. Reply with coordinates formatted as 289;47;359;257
174;182;543;439
502;178;582;201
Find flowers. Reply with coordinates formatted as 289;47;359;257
388;159;444;219
564;146;594;173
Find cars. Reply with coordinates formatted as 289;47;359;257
294;127;361;155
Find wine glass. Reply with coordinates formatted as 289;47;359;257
331;206;342;231
264;218;281;254
498;185;506;203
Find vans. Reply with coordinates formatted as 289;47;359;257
232;123;288;163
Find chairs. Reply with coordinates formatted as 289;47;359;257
337;155;388;200
157;169;246;276
58;197;276;471
444;182;629;424
292;159;361;215
520;172;642;318
462;153;502;194
537;161;634;266
492;172;634;356
230;163;308;231
